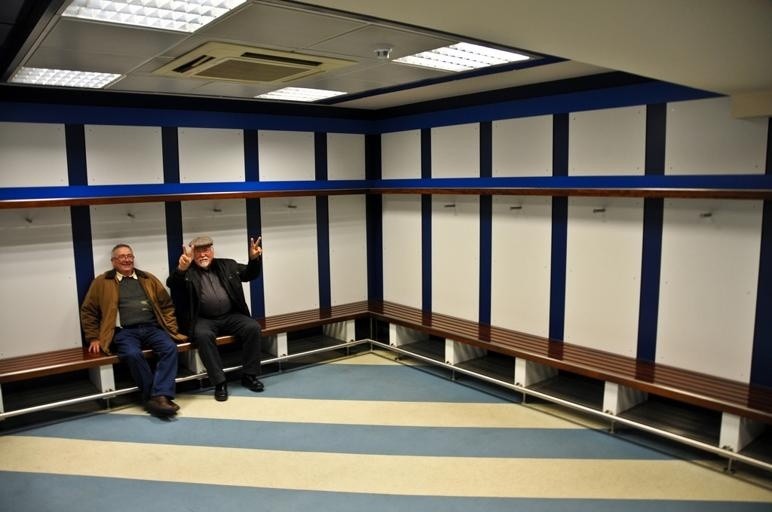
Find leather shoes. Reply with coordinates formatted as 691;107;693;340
214;380;227;401
145;395;179;414
241;373;264;390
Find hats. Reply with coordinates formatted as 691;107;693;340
189;236;213;246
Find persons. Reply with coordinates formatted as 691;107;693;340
166;236;264;401
81;244;189;418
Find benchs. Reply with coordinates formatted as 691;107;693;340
372;298;772;483
1;299;370;484
